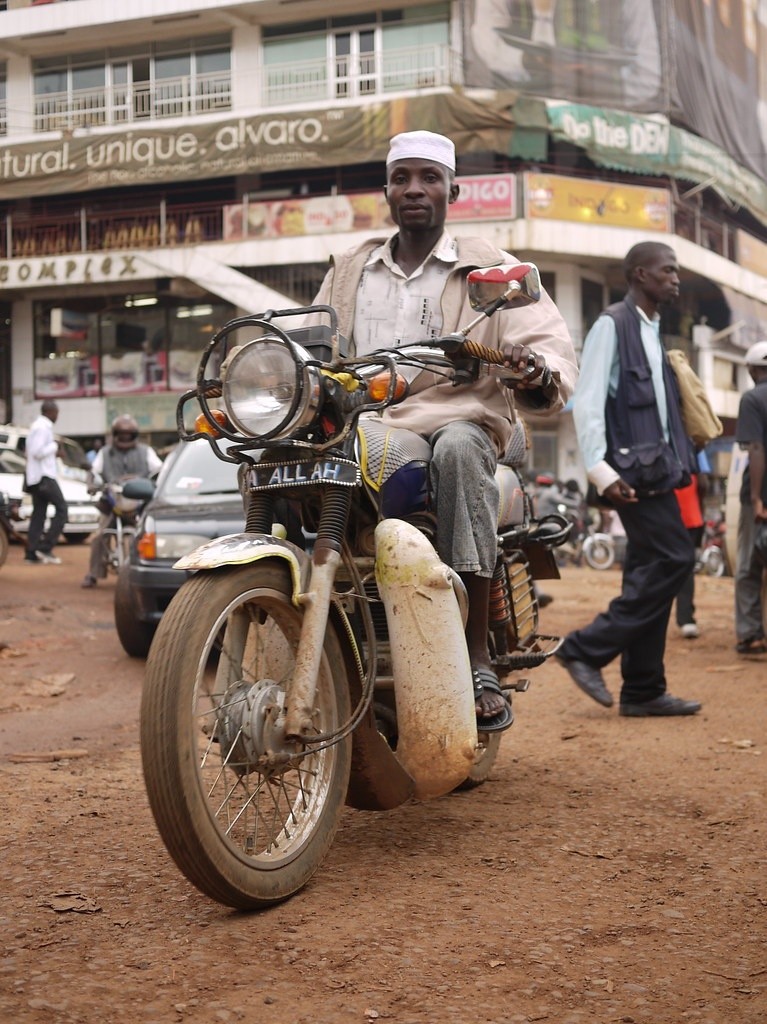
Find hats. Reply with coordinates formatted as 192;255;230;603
385;129;456;172
744;341;767;367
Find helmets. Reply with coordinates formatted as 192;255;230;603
110;415;139;449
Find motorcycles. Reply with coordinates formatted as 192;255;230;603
93;476;145;574
140;262;578;909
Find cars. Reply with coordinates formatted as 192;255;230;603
114;432;327;661
0;452;104;546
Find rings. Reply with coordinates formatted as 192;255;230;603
519;343;524;348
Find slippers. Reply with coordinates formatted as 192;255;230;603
470;667;515;732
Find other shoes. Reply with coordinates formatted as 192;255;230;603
619;694;701;716
737;639;766;653
82;576;97;588
24;553;41;563
555;640;613;707
681;624;699;638
36;549;61;565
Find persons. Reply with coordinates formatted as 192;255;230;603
733;340;767;660
574;240;707;715
674;396;709;639
521;469;611;568
297;128;576;735
82;414;165;589
85;438;103;470
25;402;68;563
